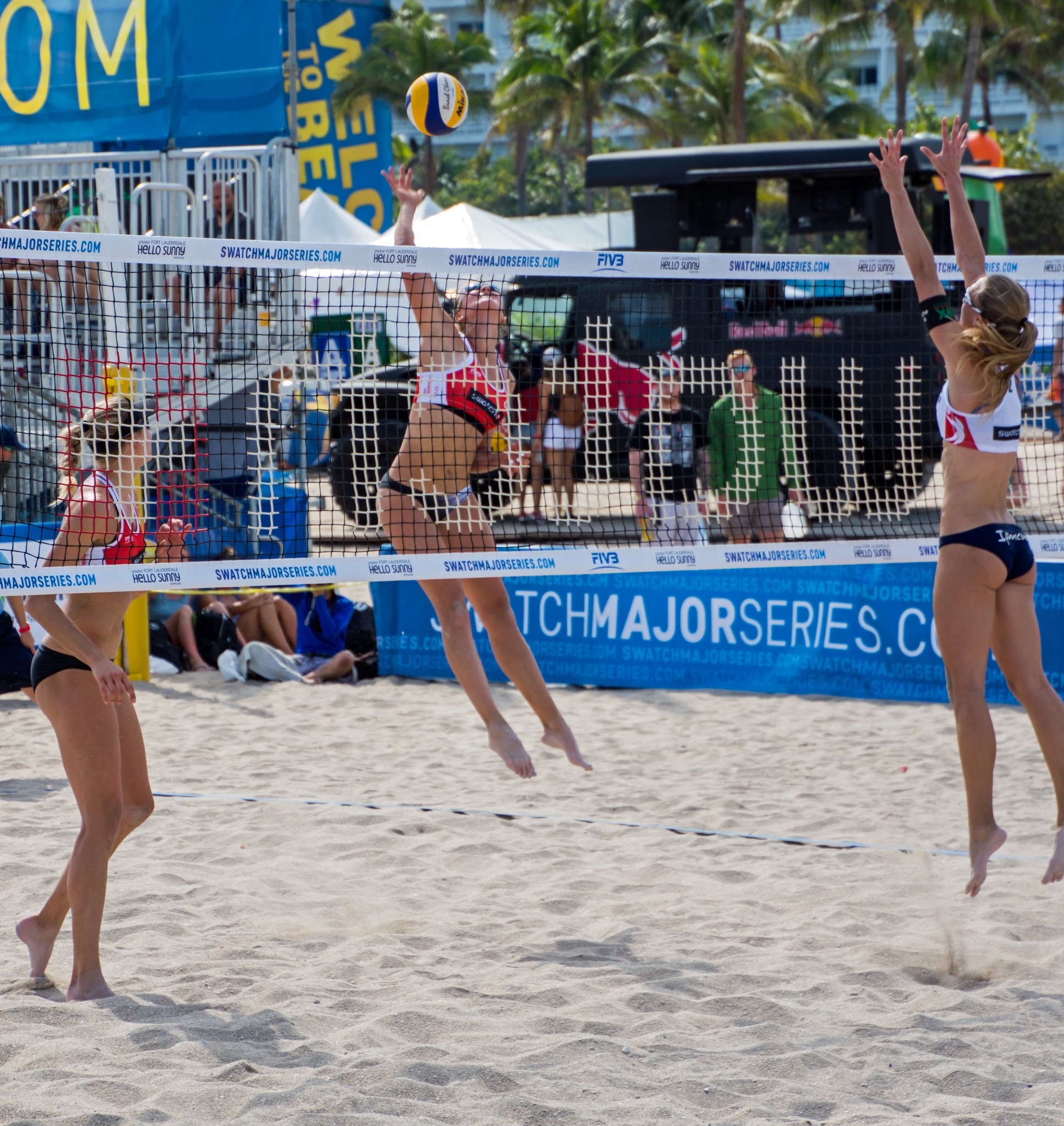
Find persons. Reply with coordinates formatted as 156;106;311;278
376;165;595;779
0;395;192;1000
868;112;1064;900
626;349;804;548
162;182;255;359
149;548;359;686
0;194;99;335
507;346;582;525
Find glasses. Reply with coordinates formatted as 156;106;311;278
457;283;500;312
728;362;752;374
34;210;45;217
118;417;150;441
962;286;982;314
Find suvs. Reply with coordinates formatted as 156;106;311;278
327;130;1055;529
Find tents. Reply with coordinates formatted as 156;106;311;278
298;185;574;359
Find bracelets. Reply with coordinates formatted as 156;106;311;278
920;294;956;333
636;493;646;503
18;623;30;635
698;494;707;498
535;435;542;438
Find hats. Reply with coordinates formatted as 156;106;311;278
542;347;562;366
656;367;677;377
88;396;157;453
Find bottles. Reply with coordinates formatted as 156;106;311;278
781;499;808;540
640;513;653;541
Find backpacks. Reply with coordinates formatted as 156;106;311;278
557;391;586;429
194;607;236;668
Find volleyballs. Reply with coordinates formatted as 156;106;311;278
405;71;468;137
